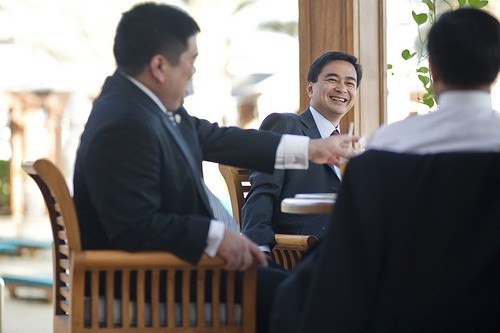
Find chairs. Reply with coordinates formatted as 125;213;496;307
22;158;260;333
216;161;318;273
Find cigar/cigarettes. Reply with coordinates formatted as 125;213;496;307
347;121;354;149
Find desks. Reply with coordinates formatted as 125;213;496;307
281;193;339;214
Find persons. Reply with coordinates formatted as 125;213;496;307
72;1;364;333
238;51;364;270
364;6;500;156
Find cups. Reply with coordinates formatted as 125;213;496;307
339;148;365;180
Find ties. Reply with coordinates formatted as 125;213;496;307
166;110;240;233
332;129;339;136
